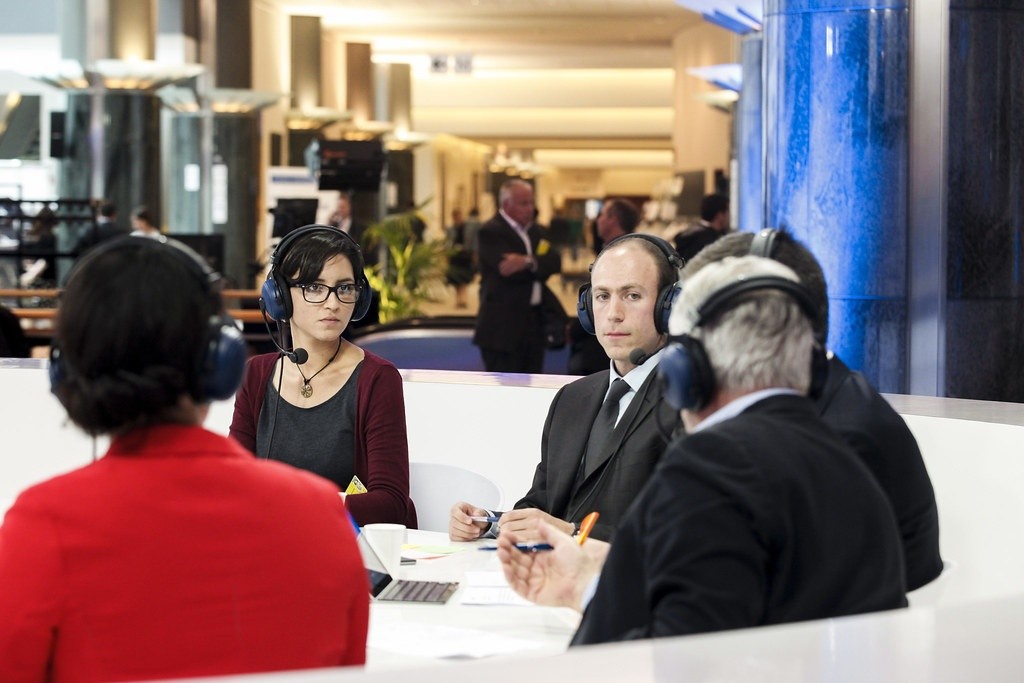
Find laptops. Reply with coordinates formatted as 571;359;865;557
346;513;461;605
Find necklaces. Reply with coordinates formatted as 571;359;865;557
296;335;341;398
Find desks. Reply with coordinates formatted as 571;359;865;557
367;527;584;659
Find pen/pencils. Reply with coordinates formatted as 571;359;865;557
470;516;500;523
578;511;598;545
479;543;553;552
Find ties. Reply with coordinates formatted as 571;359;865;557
584;377;630;478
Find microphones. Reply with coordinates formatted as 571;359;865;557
259;297;309;365
630;345;666;367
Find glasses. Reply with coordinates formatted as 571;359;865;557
288;283;363;304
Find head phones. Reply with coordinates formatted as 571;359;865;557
655;274;830;414
261;224;372;322
577;234;686;335
48;232;247;400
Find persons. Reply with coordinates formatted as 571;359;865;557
598;199;641;239
675;193;732;262
551;207;603;261
78;203;161;263
445;208;484;307
474;180;560;373
498;255;911;647
0;234;370;683
449;232;682;542
16;208;59;307
680;226;943;592
226;225;417;529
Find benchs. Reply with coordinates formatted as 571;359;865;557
0;357;1024;682
